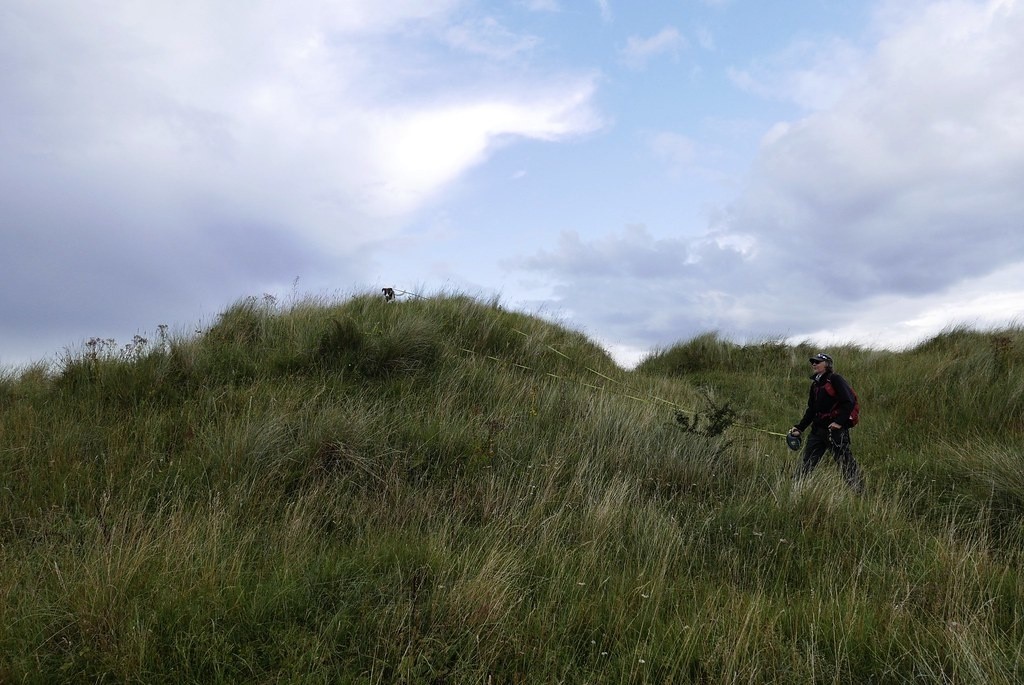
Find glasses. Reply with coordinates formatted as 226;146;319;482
811;360;824;364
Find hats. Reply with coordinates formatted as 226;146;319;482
809;353;833;365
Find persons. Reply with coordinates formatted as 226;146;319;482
789;354;865;497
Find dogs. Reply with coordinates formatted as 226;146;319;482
381;288;395;302
